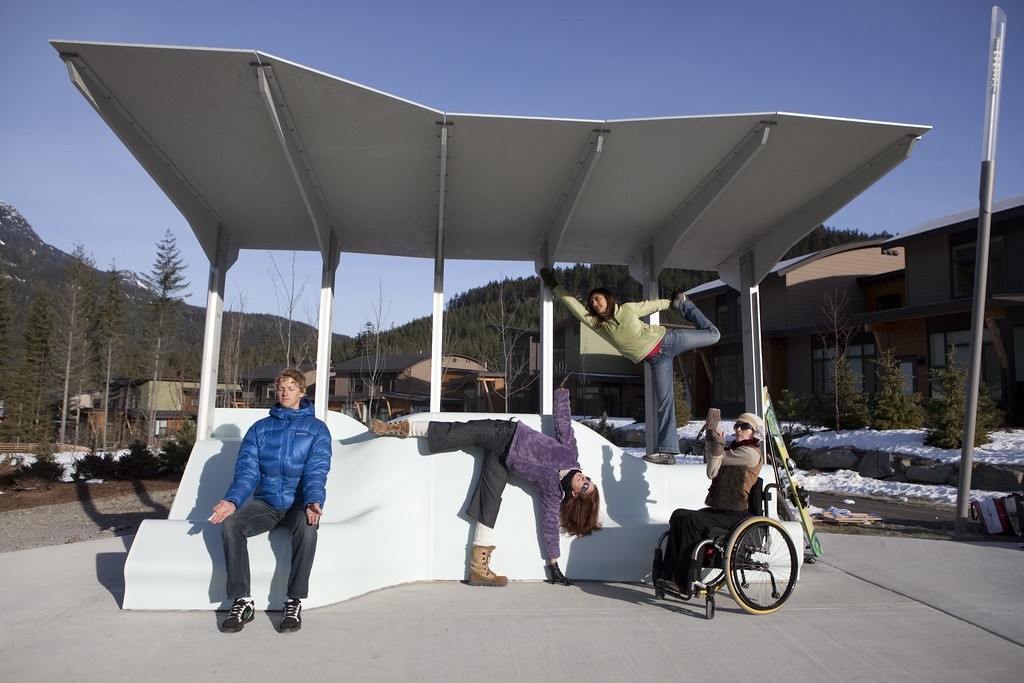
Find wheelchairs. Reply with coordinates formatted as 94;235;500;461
650;476;798;621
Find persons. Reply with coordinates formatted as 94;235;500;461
540;267;721;465
368;361;602;587
656;408;764;600
210;370;333;633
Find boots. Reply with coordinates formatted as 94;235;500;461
368;418;410;439
469;545;509;587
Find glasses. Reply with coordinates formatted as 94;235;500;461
577;476;591;500
734;423;755;434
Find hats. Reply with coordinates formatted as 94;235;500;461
735;412;765;443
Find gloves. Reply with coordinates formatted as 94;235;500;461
554;360;573;388
539;268;559;290
550;561;574;586
671;289;682;304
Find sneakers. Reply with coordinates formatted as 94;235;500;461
221;598;255;632
642;453;675;465
671;286;686;310
279;598;302;633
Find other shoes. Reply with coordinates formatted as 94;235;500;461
656;574;689;595
